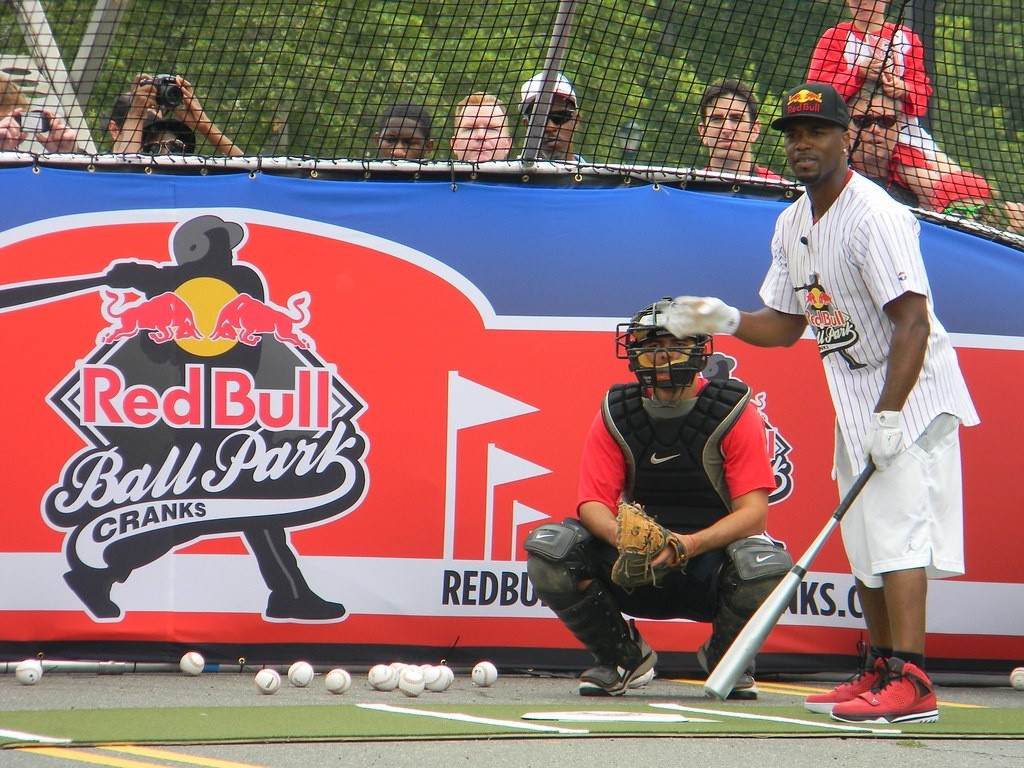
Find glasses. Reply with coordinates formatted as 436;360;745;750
142;139;185;154
525;110;575;125
848;114;898;129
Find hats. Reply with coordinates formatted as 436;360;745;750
771;81;849;132
141;118;196;156
518;70;578;119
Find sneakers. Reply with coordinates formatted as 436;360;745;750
698;633;755;689
830;647;939;724
579;620;658;695
804;630;893;714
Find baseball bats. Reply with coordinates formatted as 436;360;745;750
703;460;878;703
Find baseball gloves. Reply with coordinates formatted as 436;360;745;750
611;497;686;588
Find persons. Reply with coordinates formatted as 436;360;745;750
700;80;788;181
119;74;244;156
0;73;79;153
807;0;940;209
518;73;585;163
451;92;512;160
930;170;1024;235
667;81;980;724
374;101;434;159
523;296;793;699
842;79;919;208
109;92;162;154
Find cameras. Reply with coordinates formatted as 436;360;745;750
15;112;49;133
140;74;184;107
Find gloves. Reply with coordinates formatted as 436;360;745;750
665;295;740;340
863;410;904;471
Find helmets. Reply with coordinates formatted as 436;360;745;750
625;296;708;387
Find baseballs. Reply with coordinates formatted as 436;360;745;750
287;660;315;688
180;652;205;677
324;668;352;695
15;658;43;685
471;660;498;688
254;669;281;695
367;662;456;697
